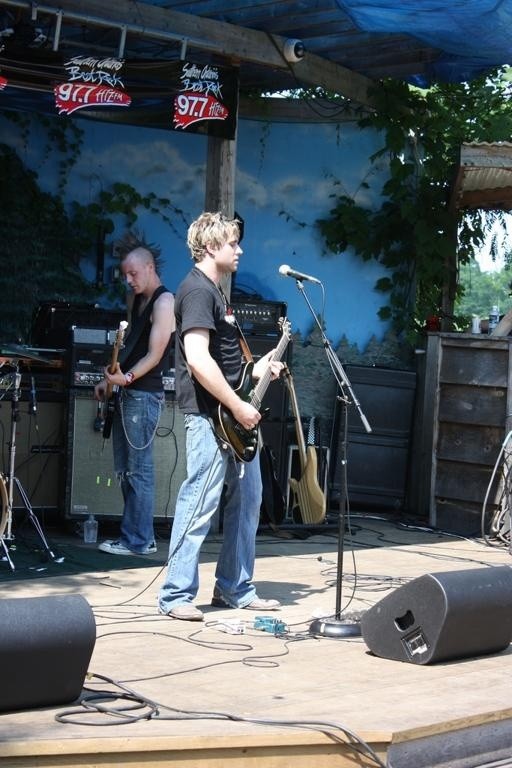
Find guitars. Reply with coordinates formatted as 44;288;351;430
213;317;292;464
281;361;327;526
92;321;128;439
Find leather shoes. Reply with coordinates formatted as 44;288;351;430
212;584;281;610
168;601;204;620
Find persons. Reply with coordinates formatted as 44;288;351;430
158;213;285;621
94;247;176;555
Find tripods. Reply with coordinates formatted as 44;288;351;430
2;372;54;558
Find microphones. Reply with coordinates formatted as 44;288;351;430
279;264;321;286
29;377;36;418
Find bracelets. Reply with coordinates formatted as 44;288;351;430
124;372;134;386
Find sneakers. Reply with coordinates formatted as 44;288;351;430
98;539;158;555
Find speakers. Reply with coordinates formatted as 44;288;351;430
219;333;294;534
0;594;96;715
361;565;512;665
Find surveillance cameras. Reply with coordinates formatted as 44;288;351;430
283;38;306;63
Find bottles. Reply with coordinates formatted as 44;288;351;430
83;514;98;544
489;304;500;335
471;315;481;336
